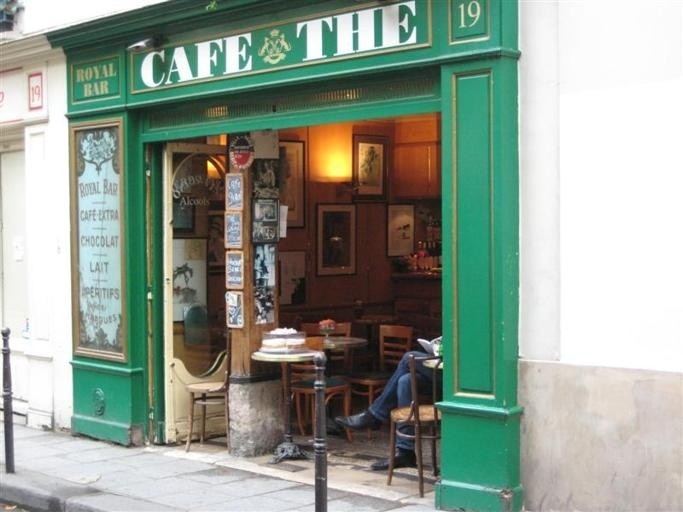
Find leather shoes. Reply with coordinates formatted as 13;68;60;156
334;410;381;433
371;450;416;472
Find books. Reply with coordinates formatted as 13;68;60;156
415;335;442;359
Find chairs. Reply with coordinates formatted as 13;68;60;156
299;322;353;417
282;336;354;444
387;354;443;497
186;328;239;454
345;324;413;441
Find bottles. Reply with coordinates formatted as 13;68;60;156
417;240;442;272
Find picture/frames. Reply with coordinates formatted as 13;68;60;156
350;132;393;206
384;202;418;260
314;200;358;279
280;138;305;229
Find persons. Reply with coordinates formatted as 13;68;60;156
332;351;442;470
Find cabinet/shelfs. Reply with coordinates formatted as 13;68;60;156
387;111;441;202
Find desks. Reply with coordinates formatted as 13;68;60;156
303;335;368;436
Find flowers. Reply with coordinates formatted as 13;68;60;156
319;319;337;339
